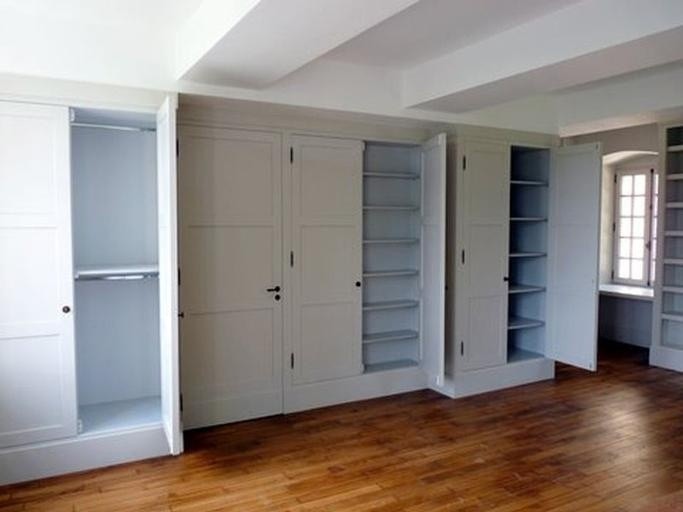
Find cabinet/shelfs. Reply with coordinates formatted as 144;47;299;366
178;117;284;432
0;73;184;487
650;110;683;374
284;128;447;418
428;132;602;398
